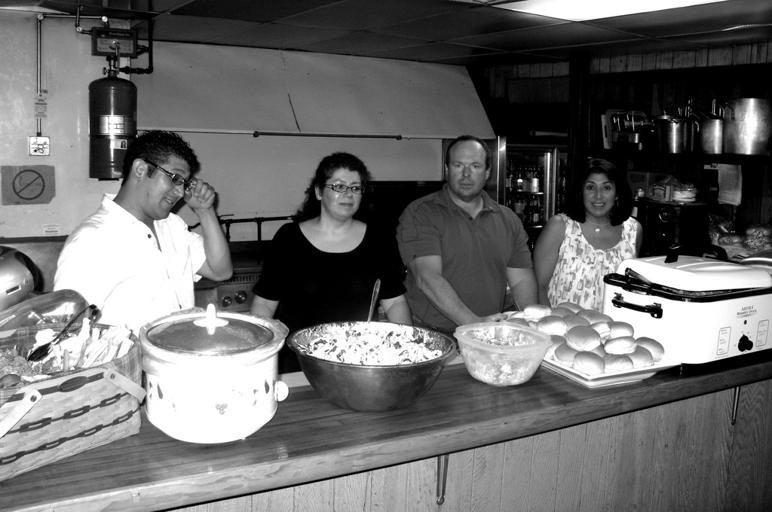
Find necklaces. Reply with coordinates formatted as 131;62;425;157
584;217;612;234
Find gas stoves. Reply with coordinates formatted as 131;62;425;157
195;253;268;311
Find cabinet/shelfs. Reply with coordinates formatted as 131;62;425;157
505;191;544;230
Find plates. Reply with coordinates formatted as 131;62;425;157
672;191;696;202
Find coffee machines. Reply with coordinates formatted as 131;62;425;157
610;111;651;154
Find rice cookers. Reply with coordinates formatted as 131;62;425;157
135;302;289;446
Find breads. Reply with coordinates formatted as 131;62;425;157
508;302;665;377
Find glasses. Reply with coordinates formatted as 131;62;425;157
145;159;190;187
324;184;362;193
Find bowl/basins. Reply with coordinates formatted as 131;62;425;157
449;320;553;387
286;322;460;414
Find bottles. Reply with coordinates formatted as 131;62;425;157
501;152;547;228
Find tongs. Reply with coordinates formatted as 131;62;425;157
28;303;102;360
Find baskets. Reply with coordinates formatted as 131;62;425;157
0;315;150;485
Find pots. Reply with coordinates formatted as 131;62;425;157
723;97;770;158
697;115;726;156
652;116;701;154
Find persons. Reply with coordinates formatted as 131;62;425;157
525;156;646;318
243;151;412;378
394;136;539;332
54;129;235;342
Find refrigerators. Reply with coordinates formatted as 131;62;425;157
440;130;573;254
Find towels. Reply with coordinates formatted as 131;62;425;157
717;163;742;206
